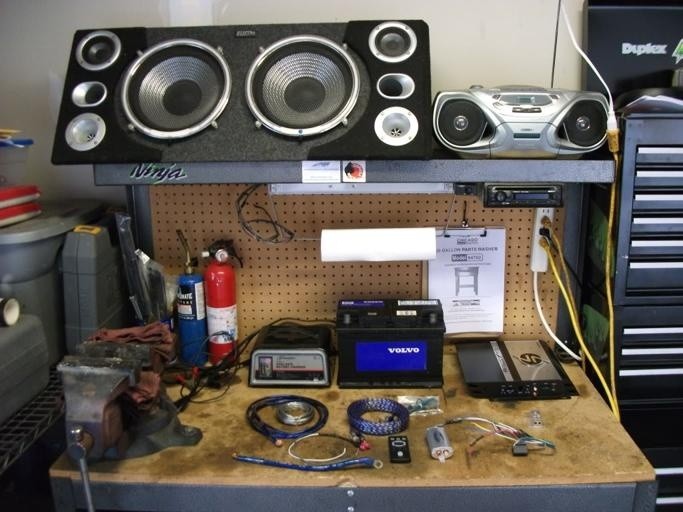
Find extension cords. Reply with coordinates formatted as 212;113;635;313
530;207;554;273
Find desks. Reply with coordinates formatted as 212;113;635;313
48;349;658;512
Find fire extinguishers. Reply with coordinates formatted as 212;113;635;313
201;239;243;368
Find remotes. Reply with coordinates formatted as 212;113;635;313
389;435;410;463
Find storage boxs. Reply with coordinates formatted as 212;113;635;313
0;314;49;422
0;197;109;358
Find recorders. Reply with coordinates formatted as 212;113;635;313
433;85;612;156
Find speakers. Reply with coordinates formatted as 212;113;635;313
51;17;431;165
